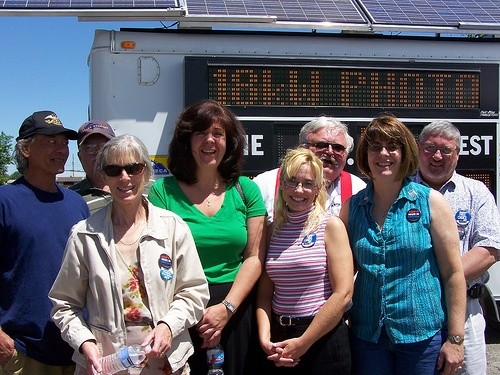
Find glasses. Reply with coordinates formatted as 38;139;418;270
301;141;346;156
283;179;318;190
33;138;69;146
421;144;458;155
80;144;102;154
101;163;147;178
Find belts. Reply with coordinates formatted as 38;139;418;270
270;311;315;327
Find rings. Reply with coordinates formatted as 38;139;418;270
458;366;462;370
161;352;164;357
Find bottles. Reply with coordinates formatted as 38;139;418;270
206;343;225;375
91;344;152;375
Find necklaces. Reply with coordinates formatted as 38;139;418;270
195;183;220;207
113;218;144;245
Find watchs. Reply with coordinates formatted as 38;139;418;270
447;335;464;346
222;300;238;314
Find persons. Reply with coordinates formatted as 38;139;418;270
408;120;500;375
49;134;210;375
0;111;92;375
253;117;368;230
340;116;467;375
68;120;155;216
148;100;269;375
255;147;354;375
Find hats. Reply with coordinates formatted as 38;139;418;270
77;118;116;147
15;111;79;141
